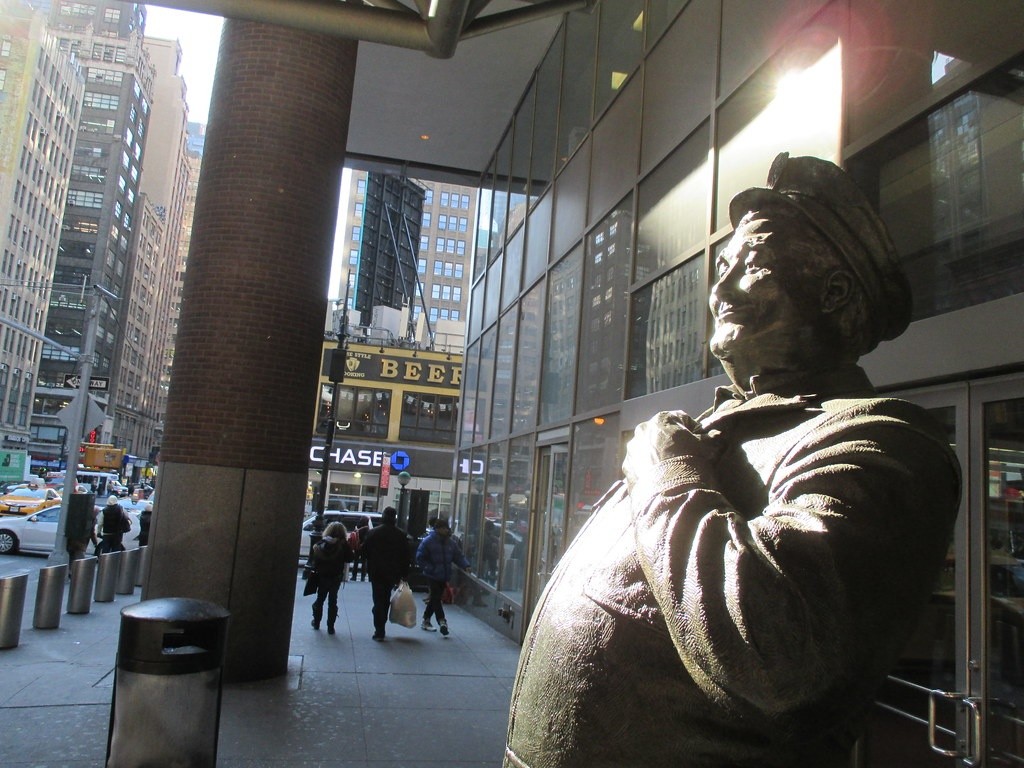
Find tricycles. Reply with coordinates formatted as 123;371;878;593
131;485;154;504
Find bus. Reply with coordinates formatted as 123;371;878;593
60;470;128;498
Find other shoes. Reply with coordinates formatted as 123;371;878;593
423;595;429;604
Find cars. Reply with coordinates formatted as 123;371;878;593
0;484;62;517
452;517;522;569
0;504;141;558
43;472;79;496
300;509;409;571
0;481;39;496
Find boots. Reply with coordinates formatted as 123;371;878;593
311;604;323;630
327;606;339;634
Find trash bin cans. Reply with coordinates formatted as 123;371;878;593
104;594;231;768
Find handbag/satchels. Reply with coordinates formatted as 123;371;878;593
442;587;454;604
389;580;417;628
121;513;133;532
303;573;320;597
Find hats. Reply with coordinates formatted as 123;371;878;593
145;504;153;511
107;495;117;505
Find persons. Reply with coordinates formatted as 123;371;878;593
303;505;472;641
499;150;962;768
483;520;529;588
69;475;153;579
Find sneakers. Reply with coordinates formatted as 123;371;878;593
421;617;437;632
438;619;449;636
372;634;385;642
